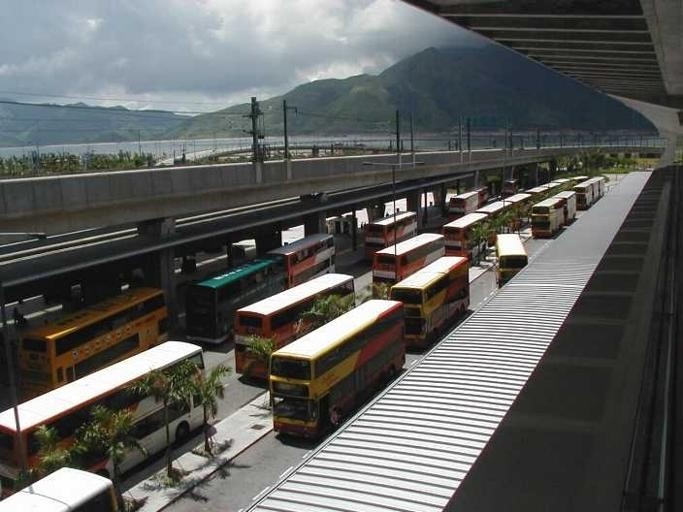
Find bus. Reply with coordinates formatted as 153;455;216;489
233;273;357;381
370;232;446;286
185;253;288;344
0;340;209;497
494;233;529;289
267;297;409;447
388;256;471;354
364;211;418;248
262;232;337;290
15;285;170;401
0;468;119;511
440;175;603;265
